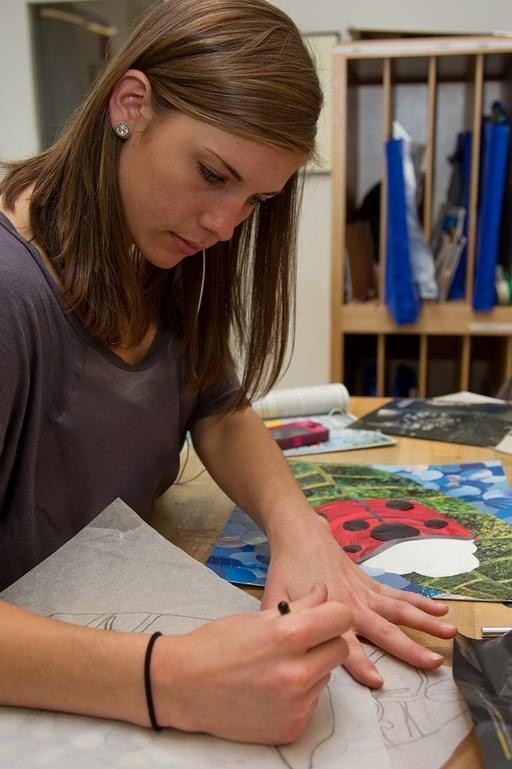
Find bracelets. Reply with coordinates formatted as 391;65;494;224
144;632;162;732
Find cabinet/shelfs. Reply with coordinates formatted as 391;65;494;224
328;37;512;403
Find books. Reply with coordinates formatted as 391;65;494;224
241;383;396;459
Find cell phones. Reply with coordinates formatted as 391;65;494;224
267;420;329;450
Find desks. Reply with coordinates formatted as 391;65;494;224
147;395;512;769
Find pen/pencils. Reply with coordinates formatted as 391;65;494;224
278;601;290;615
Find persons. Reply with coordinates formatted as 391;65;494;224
0;0;459;746
